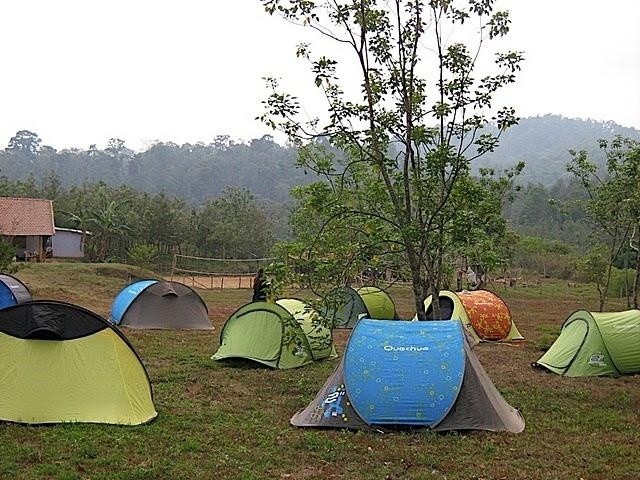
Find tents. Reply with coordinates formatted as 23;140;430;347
0;300;159;427
410;289;525;344
289;316;526;434
531;307;640;377
211;297;338;370
0;273;33;309
110;279;215;331
320;285;398;329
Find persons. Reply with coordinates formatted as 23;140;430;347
252;269;271;301
